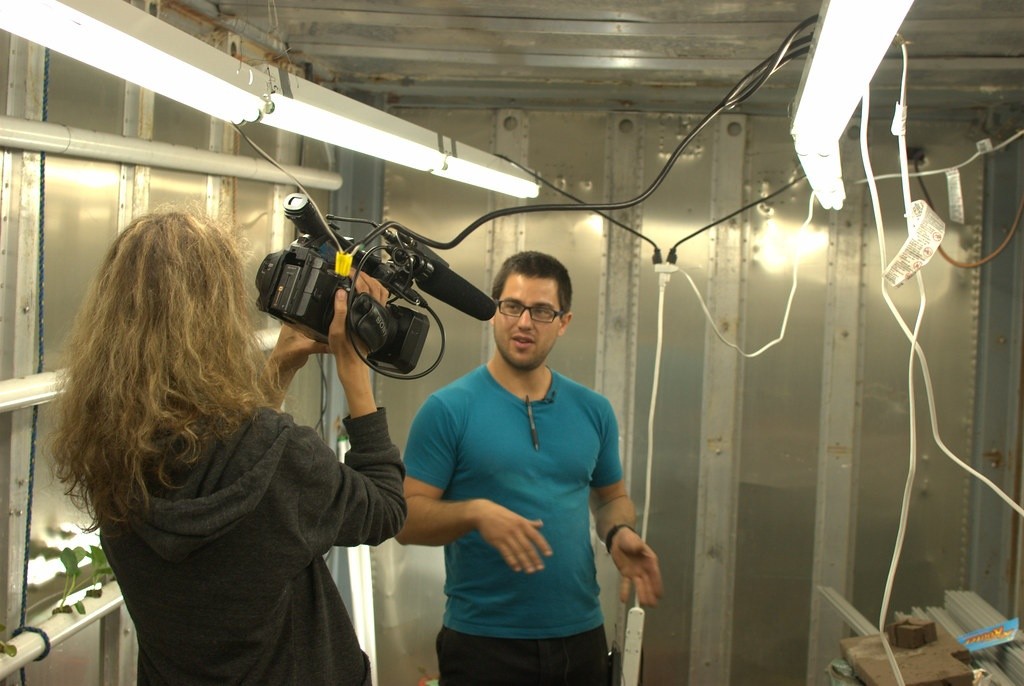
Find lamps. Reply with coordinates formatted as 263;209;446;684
262;71;543;199
789;0;914;211
0;0;266;128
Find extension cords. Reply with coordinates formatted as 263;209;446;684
620;608;645;686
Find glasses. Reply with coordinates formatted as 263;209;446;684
494;299;564;323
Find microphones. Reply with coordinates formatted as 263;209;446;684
416;259;497;323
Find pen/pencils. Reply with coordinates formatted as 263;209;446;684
526;394;539;452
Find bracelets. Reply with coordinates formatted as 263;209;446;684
606;523;640;554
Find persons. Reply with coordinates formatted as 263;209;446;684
44;208;408;686
394;250;710;686
